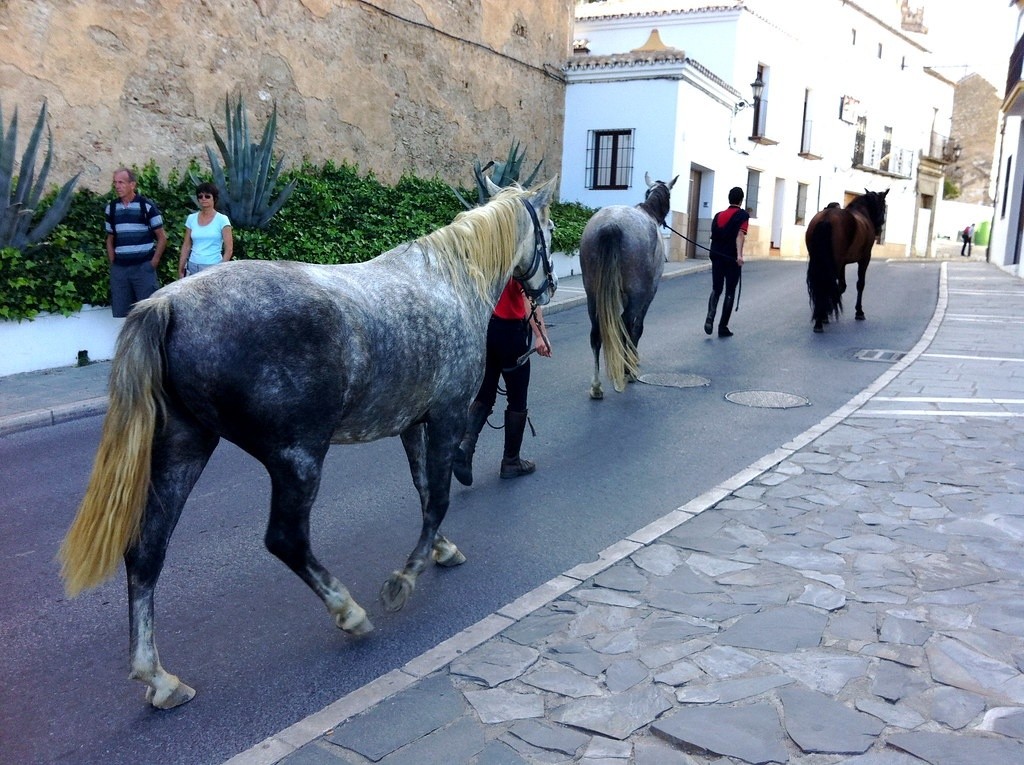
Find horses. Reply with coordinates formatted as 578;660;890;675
50;173;559;712
805;187;892;334
579;171;681;400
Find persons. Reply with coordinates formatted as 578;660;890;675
704;186;750;337
453;273;553;487
178;184;233;280
823;202;842;211
103;167;166;318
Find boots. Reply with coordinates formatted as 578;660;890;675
500;409;535;480
718;296;735;338
705;290;720;335
453;402;491;486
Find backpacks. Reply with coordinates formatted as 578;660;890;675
962;227;970;240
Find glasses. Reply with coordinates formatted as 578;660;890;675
198;195;214;199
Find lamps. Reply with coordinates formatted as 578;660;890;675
945;145;962;163
739;77;764;108
